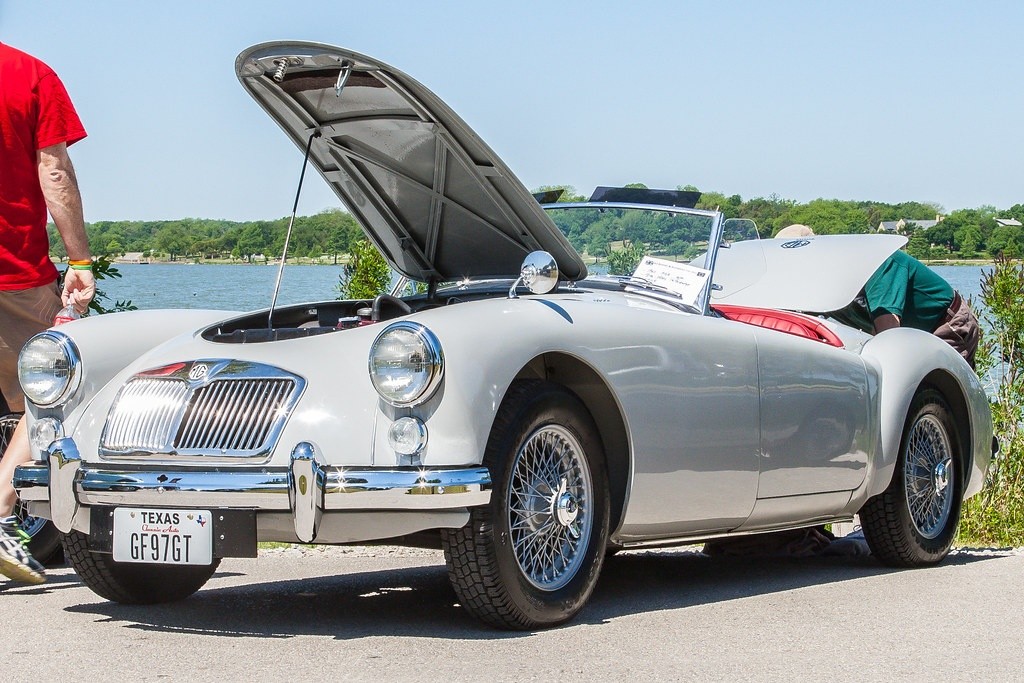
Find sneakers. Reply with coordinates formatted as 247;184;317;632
0;515;47;584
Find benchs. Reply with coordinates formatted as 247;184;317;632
710;303;843;348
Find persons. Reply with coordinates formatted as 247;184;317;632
0;43;96;584
774;224;999;463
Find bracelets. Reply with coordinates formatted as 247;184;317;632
67;259;94;271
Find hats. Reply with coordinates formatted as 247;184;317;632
773;224;816;238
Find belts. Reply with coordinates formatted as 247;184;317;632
934;290;961;331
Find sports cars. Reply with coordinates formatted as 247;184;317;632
12;38;995;630
